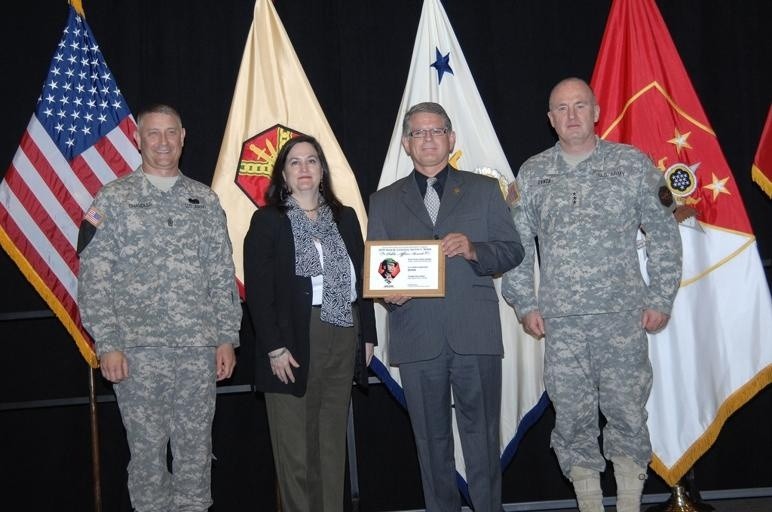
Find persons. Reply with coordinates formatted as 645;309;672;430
368;98;527;511
244;135;381;511
74;102;245;510
381;258;398;280
504;78;685;511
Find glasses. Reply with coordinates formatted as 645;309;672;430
407;126;449;139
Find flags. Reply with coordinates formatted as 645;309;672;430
207;0;368;307
0;0;142;373
368;0;548;509
750;106;771;204
589;1;772;493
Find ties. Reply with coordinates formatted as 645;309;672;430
424;177;440;226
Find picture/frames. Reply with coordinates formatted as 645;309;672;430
363;240;446;299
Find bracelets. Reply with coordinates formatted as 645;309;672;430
268;348;287;360
